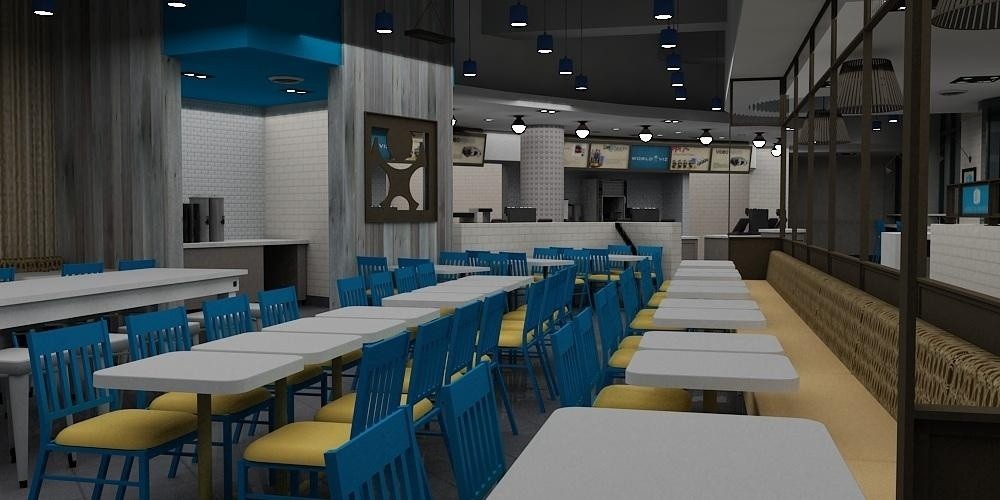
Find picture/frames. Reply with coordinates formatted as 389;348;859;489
961;167;978;183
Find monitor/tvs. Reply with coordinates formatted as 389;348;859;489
769;218;778;228
731;219;749;233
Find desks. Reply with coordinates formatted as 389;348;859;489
491;406;869;500
883;223;897;230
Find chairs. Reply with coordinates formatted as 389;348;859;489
895;218;903;233
871;218;886;264
1;243;998;500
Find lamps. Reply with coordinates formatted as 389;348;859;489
452;112;781;157
375;1;687;101
930;1;998;30
712;97;722;111
